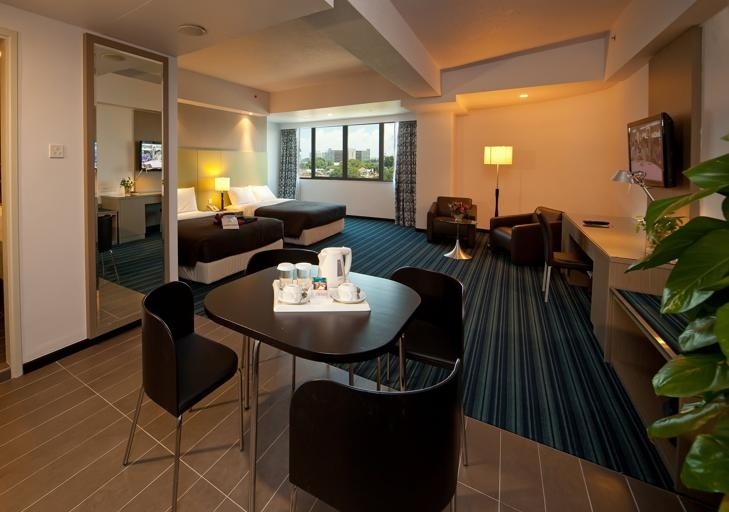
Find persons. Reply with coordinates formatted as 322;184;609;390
631;140;638;160
639;137;648;160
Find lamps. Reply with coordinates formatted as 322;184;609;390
214;178;231;212
483;145;513;218
613;170;682;236
177;25;207;40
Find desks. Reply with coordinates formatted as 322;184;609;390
562;210;711;359
100;193;163;248
436;215;478;261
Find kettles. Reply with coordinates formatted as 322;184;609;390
317;246;352;288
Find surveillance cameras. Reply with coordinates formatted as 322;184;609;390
250;91;262;102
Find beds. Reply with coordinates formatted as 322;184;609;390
176;187;286;285
222;185;346;247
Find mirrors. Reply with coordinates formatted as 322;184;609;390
82;31;170;342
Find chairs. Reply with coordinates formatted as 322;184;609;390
122;281;245;511
239;247;331;411
387;265;469;371
287;356;467;512
536;209;594;303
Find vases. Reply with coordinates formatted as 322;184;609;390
453;212;464;223
125;189;133;197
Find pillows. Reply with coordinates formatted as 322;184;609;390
250;184;277;202
175;187;200;213
227;187;251;205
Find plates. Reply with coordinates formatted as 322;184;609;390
331;291;367;304
277;294;307;305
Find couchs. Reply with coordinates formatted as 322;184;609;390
489;205;565;267
426;196;478;249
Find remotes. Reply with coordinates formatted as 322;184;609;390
582;220;610;227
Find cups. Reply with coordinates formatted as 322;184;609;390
337;283;357;301
276;263;293;289
295;263;311;288
281;284;301;301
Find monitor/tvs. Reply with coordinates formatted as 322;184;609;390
627;111;676;188
138;140;162;172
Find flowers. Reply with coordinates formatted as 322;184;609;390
120;176;134;186
448;201;471;215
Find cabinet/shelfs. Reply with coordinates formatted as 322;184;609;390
608;286;729;506
